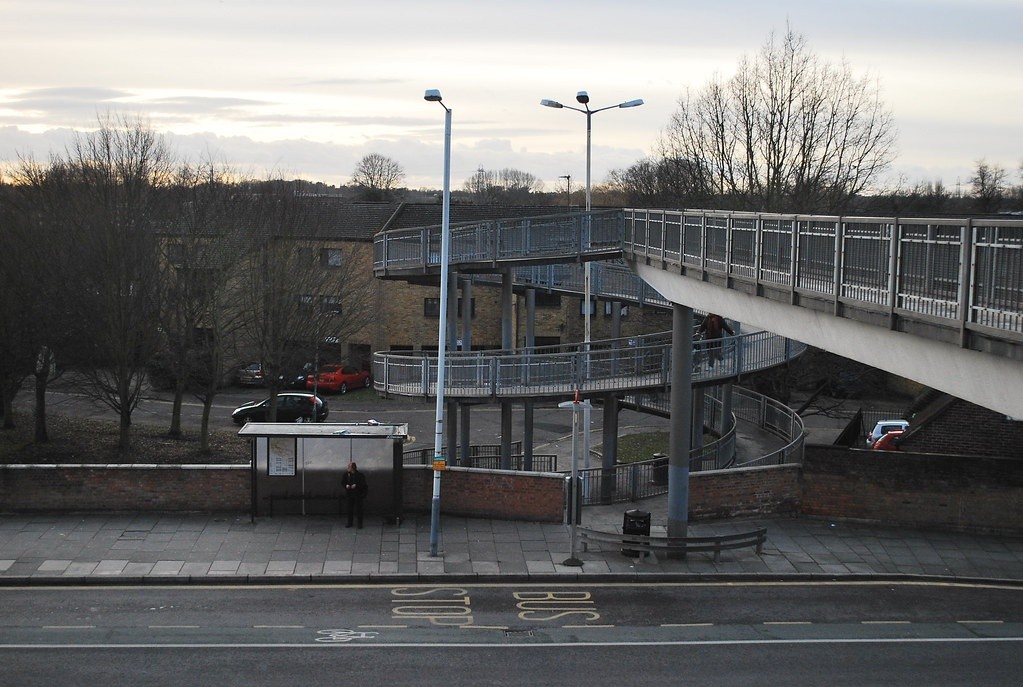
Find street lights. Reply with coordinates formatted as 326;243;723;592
424;89;453;559
558;175;571;213
540;91;645;502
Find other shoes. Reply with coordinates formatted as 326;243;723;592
357;522;363;529
345;522;352;528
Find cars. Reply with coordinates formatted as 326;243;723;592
306;364;371;395
874;429;906;451
279;367;306;390
557;401;587;409
236;363;272;388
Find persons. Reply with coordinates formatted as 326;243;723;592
696;313;735;370
342;462;368;529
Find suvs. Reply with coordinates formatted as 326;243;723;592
866;419;910;446
232;393;329;426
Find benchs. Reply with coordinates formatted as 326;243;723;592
263;493;364;517
